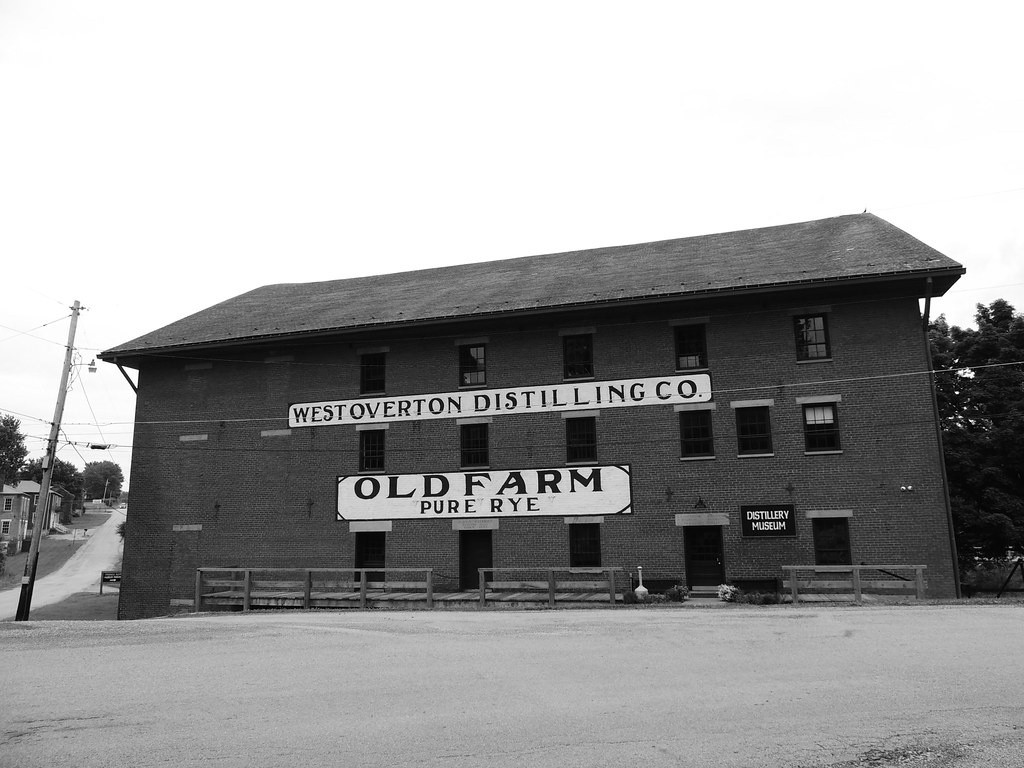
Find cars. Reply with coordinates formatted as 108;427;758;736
120;503;127;509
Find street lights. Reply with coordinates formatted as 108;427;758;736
14;358;98;621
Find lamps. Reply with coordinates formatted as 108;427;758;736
694;496;708;509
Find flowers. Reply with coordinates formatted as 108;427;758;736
717;585;740;601
